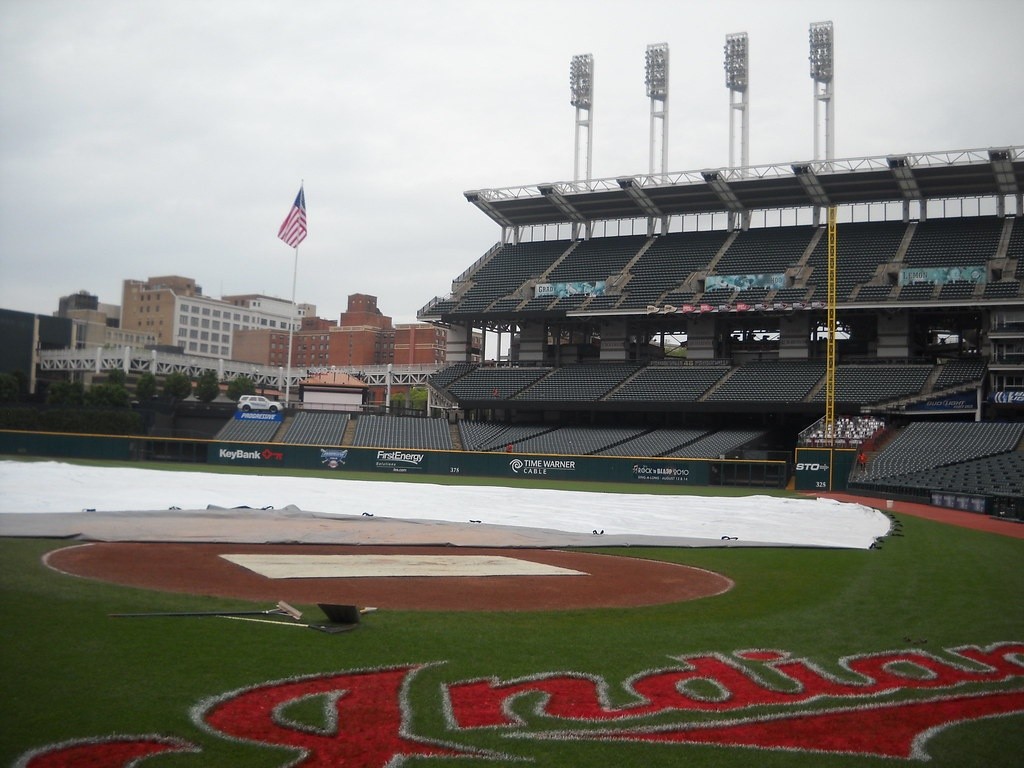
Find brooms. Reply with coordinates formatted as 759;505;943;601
108;600;303;621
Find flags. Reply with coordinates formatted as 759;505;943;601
276;187;307;248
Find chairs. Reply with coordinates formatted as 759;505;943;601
213;215;1024;497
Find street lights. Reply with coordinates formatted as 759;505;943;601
645;41;669;237
725;32;750;233
385;364;392;413
809;21;836;228
570;53;595;240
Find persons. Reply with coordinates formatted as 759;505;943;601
506;442;512;452
493;387;499;397
858;450;867;472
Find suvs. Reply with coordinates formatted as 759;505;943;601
235;393;284;413
